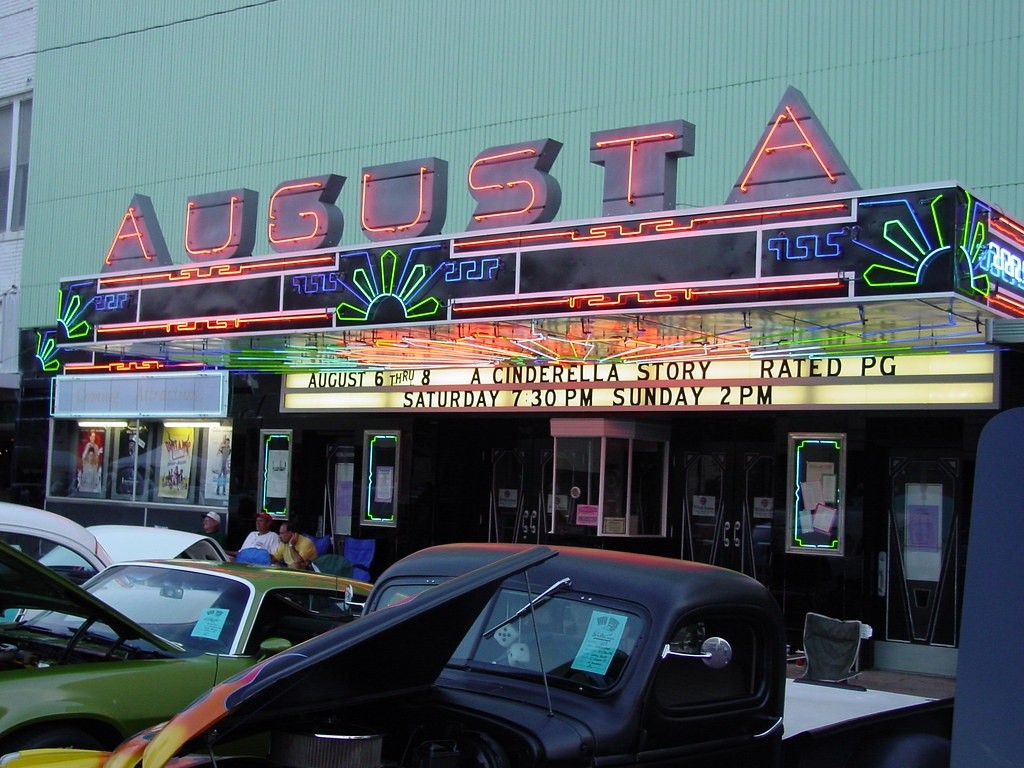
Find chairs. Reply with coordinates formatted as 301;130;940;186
235;535;376;584
799;612;862;685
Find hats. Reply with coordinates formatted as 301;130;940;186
202;511;221;525
254;512;272;525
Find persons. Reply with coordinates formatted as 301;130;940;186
168;467;184;485
82;430;103;473
198;512;225;548
226;513;282;565
272;522;318;569
217;439;230;495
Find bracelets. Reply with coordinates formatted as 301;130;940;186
289;545;294;547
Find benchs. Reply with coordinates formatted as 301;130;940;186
264;616;349;646
527;630;743;703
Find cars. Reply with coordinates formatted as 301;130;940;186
1;544;390;753
1;502;231;584
0;540;955;768
